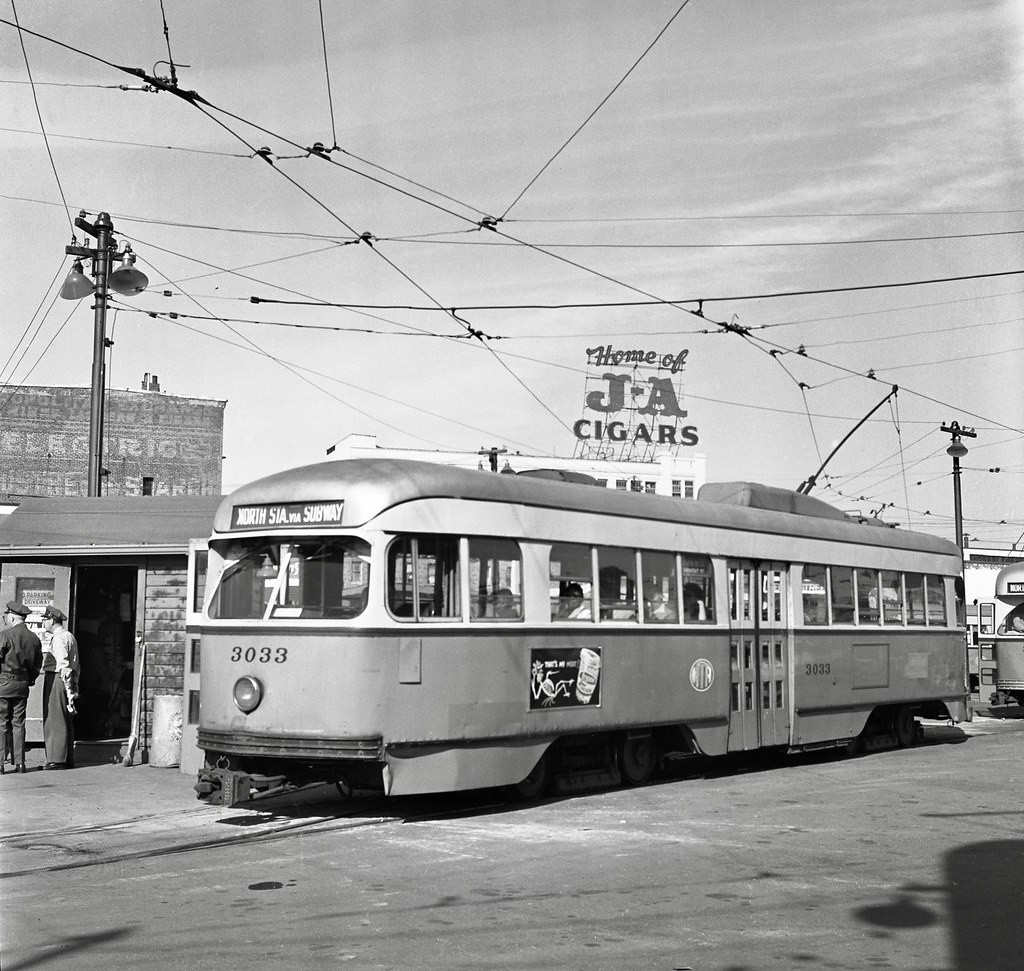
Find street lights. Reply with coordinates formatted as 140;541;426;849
62;207;151;501
941;422;978;580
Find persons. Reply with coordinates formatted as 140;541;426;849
491;589;518;617
565;582;591;618
646;584;676;621
684;582;710;622
38;605;81;771
0;600;44;775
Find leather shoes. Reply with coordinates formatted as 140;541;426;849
0;765;4;774
15;764;26;773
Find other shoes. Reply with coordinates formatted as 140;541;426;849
38;762;65;770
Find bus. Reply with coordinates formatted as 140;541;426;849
993;561;1024;704
194;387;972;813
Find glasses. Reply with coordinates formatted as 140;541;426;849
684;592;695;597
567;594;580;597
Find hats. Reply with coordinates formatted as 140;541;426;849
39;605;67;624
4;601;33;616
